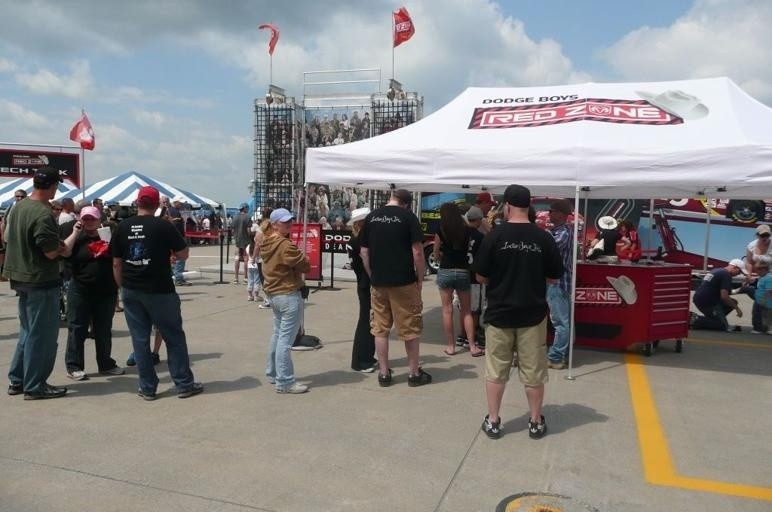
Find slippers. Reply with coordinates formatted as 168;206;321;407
444;348;456;356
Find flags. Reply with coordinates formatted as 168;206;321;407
393;7;416;48
69;108;95;151
257;23;279;56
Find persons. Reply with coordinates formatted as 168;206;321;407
254;184;772;439
0;168;204;400
185;200;272;300
258;100;415;229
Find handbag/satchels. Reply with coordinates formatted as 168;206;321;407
587;238;604;260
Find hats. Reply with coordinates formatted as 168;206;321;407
81;207;101;219
598;216;617;230
466;185;575;220
394;189;412;210
270;209;296;223
34;167;63;184
757;225;772;237
730;258;748;275
607;275;637;305
237;202;249;210
137;186;159;206
347;208;371;225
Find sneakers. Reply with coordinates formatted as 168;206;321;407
361;360;431;388
547;359;565;369
457;328;485;356
67;371;87;380
482;415;502;438
137;388;155;400
529;415;546;438
277;384;308;394
178;383;202;397
234;278;272;308
99;367;124;374
8;381;22;394
23;381;67;399
126;352;160;366
176;280;192;286
689;312;697;329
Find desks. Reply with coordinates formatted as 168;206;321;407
547;257;691;355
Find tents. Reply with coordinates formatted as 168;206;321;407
301;80;772;378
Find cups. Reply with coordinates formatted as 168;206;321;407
97;225;112;246
